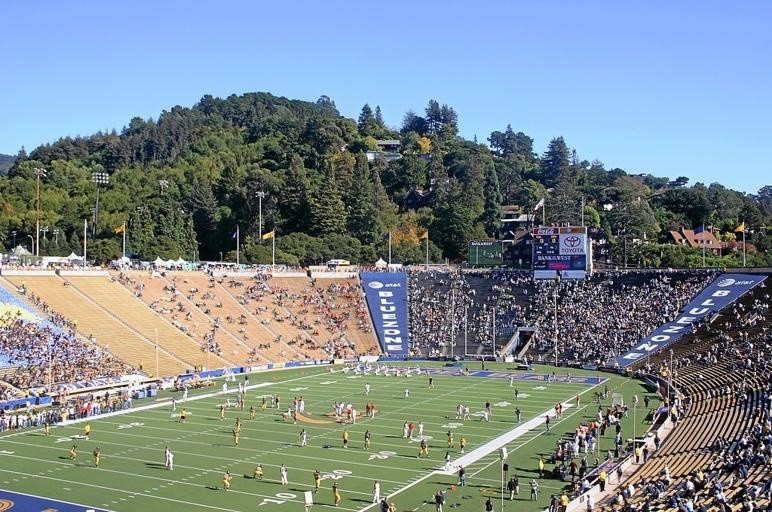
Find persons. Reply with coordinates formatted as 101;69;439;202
0;259;772;512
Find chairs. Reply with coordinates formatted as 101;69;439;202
407;269;771;368
543;369;769;512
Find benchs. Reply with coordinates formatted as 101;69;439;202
0;269;382;429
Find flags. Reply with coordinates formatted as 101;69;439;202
230;229;237;241
693;224;703;234
262;230;273;240
734;224;744;232
532;197;544;211
114;223;124;235
575;197;582;208
382;232;389;240
417;230;429;240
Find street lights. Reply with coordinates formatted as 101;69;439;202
33;165;48;255
83;219;89;268
90;207;96;237
155;327;161;377
647;340;680;420
498;446;508;512
11;225;61;257
631;394;638;454
48;337;54;393
553;331;558;368
552;294;560;322
255;190;266;239
159;179;169;194
92;170;110;224
